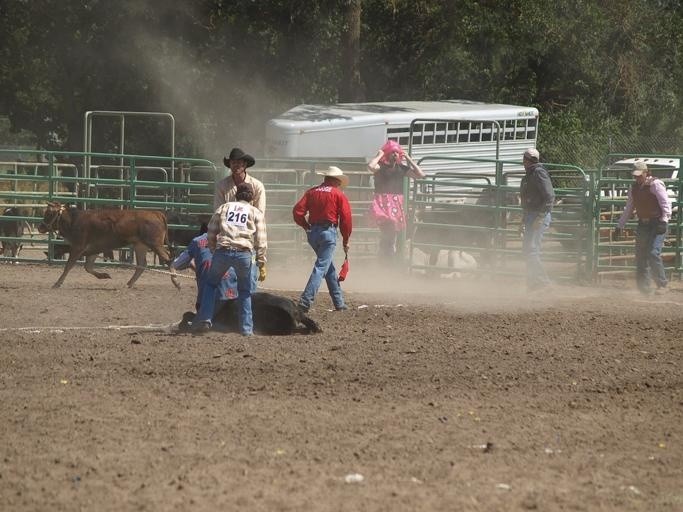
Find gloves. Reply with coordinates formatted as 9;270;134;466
613;228;622;242
656;221;667;234
530;212;545;231
258;264;267;281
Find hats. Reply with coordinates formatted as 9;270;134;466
224;148;254;167
316;166;349;186
526;148;539;163
633;162;647;177
237;183;253;193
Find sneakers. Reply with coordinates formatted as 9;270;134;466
190;322;211;330
655;285;667;296
336;305;348;311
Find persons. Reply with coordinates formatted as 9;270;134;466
614;162;672;296
519;148;556;292
292;166;352;313
174;222;238;317
215;148;266;296
191;185;267;338
368;141;425;257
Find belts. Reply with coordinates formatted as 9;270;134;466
221;248;248;253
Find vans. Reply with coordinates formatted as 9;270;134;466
585;154;680;220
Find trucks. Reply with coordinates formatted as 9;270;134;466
258;98;542;212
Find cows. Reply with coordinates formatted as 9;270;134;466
178;293;323;336
0;205;27;265
37;200;184;291
